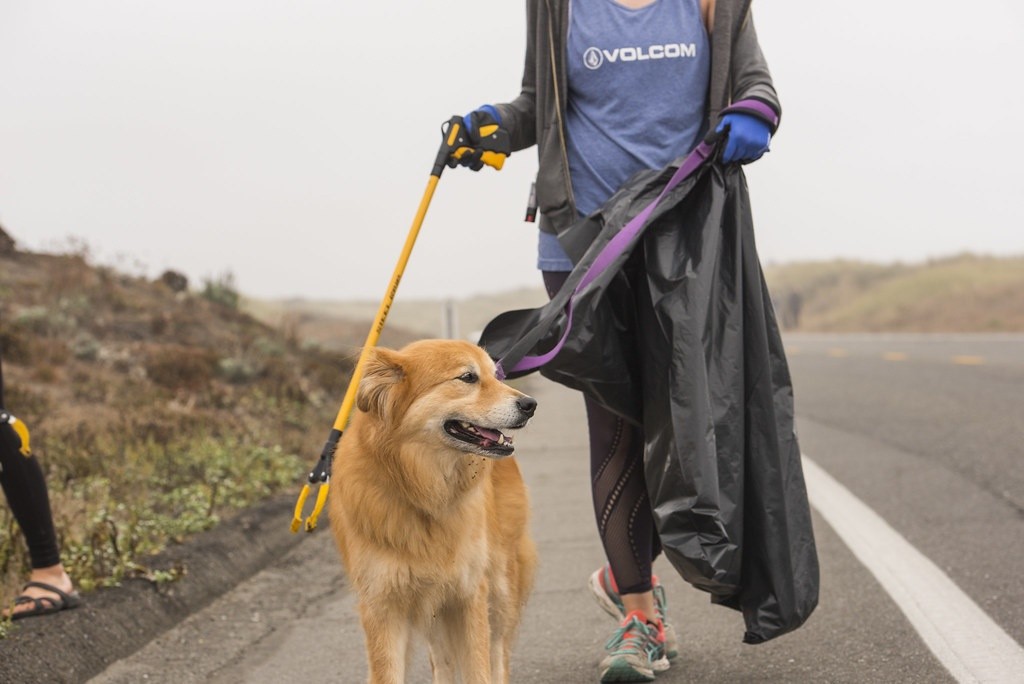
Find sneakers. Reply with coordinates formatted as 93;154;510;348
597;610;670;684
587;562;681;662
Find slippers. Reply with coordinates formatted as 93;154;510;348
9;582;84;619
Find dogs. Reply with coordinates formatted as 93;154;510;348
330;338;538;684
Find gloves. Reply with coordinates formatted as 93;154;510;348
449;104;503;172
702;111;773;168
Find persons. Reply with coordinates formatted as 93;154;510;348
0;410;80;621
448;0;783;684
